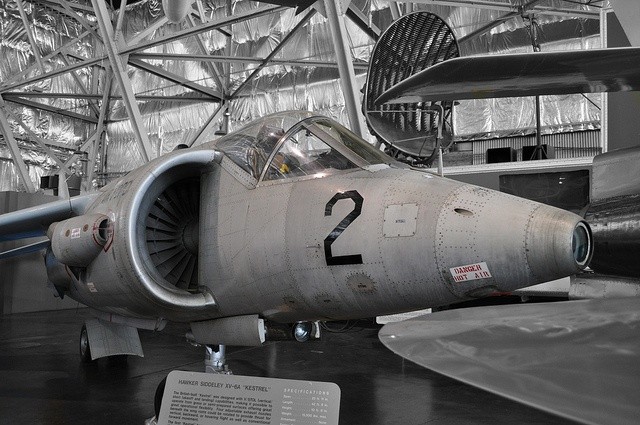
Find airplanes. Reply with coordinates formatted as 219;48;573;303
1;108;592;425
